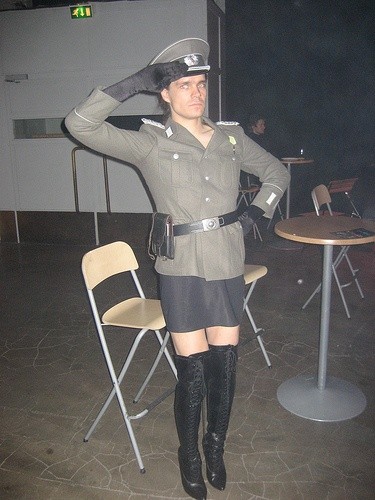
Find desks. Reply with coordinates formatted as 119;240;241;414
274;215;375;422
280;159;314;219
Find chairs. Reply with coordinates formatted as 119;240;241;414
239;174;365;368
82;241;178;474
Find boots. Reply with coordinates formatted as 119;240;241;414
206;344;238;490
171;350;209;500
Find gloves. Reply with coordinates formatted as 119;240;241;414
103;60;191;102
238;205;264;237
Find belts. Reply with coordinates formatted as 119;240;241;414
172;210;243;238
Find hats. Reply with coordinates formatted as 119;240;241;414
150;37;211;77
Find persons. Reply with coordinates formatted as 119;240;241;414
65;38;291;500
245;113;272;153
